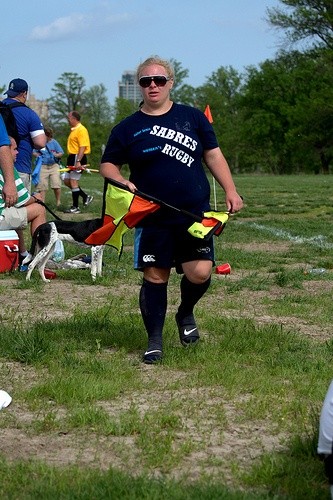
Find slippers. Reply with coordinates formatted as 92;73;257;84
142;345;163;364
176;313;200;347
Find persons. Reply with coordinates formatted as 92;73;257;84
0;135;47;266
33;127;64;207
65;109;94;213
99;56;245;367
316;378;333;484
0;113;18;208
0;78;47;196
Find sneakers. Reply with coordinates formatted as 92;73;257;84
64;206;80;214
83;195;94;208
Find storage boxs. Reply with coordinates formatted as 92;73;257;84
0;229;20;275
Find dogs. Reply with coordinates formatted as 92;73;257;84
22;218;105;282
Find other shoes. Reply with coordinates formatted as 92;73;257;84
19;254;34;266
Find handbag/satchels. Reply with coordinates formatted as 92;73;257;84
51;240;64;262
54;157;61;163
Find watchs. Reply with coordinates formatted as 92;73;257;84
76;158;82;162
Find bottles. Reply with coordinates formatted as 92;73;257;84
75;167;81;180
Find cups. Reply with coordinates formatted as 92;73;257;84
43;268;56;279
216;262;231;274
51;150;56;152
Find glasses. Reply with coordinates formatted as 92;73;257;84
139;76;170;88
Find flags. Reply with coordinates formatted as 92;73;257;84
187;209;230;241
203;103;214;123
84;177;161;260
60;166;92;175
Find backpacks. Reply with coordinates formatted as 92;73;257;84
0;102;29;150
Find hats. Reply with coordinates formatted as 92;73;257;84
3;78;28;98
